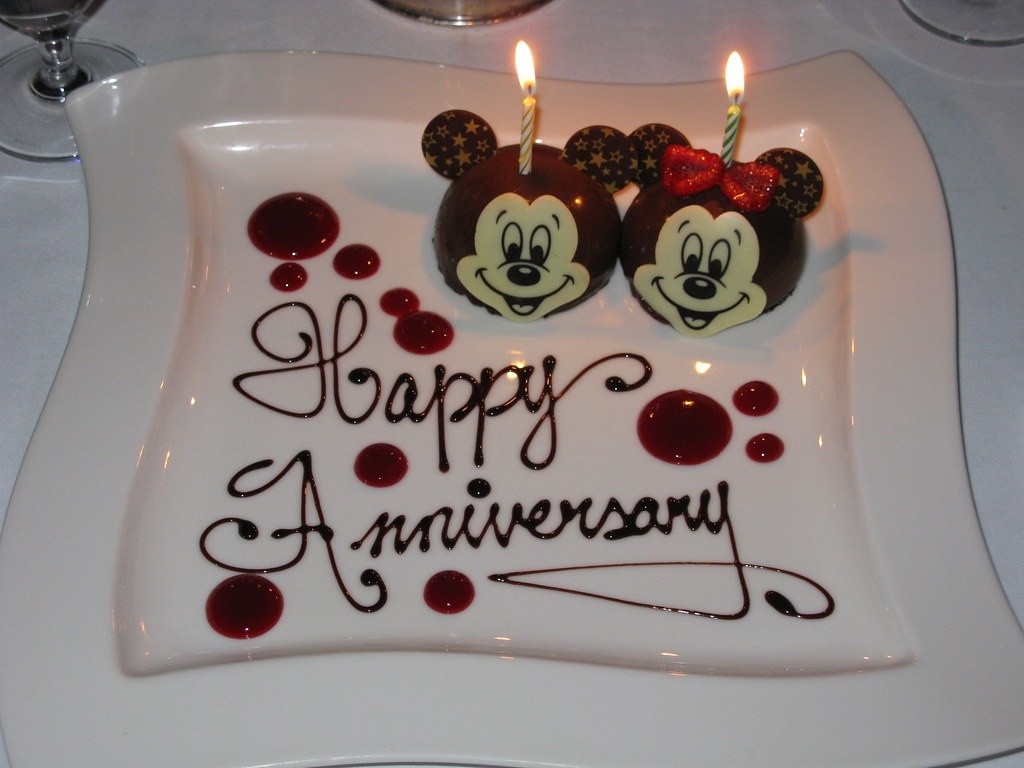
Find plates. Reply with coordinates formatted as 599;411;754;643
0;51;1024;768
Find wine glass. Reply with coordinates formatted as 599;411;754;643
0;0;145;162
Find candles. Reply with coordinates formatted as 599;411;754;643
719;50;745;169
514;39;537;175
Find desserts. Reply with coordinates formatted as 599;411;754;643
420;109;822;339
